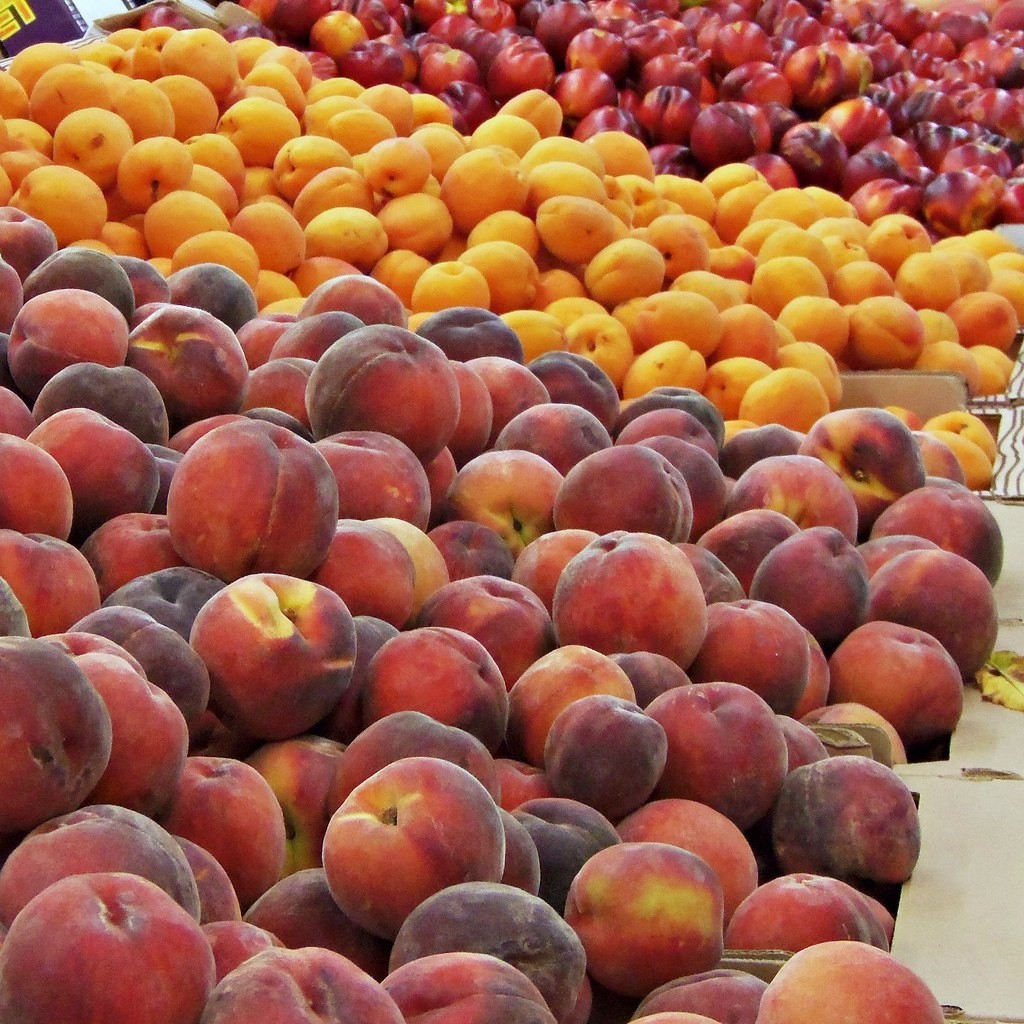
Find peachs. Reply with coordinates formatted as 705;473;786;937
0;0;1024;1024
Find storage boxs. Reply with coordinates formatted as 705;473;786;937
82;0;226;40
